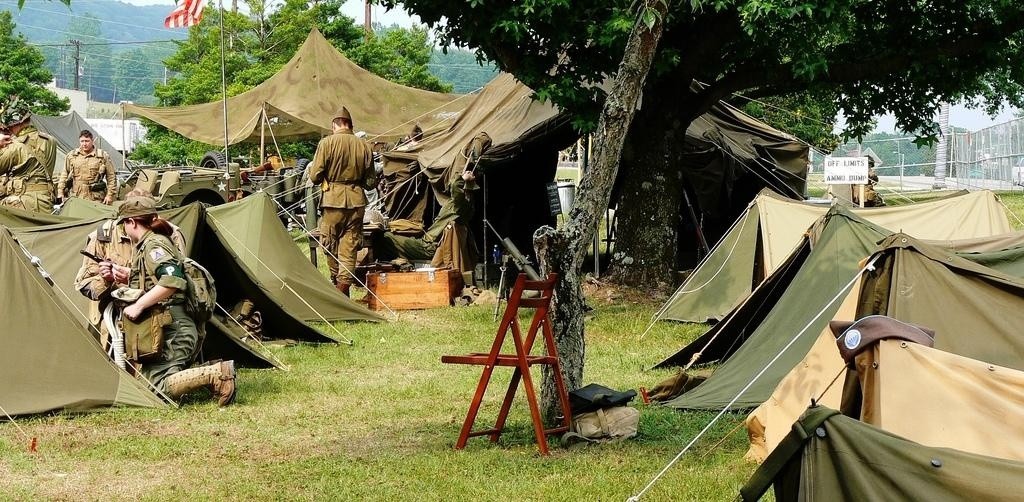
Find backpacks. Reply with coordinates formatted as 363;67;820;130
143;241;217;321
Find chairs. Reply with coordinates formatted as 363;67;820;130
442;272;572;455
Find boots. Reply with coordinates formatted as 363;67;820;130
165;359;235;408
336;282;349;297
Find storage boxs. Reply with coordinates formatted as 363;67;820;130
367;268;462;310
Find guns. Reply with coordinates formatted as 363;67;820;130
482;215;541;321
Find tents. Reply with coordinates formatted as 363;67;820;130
744;227;1024;466
11;213;288;376
827;314;1024;462
1;224;179;417
60;189;398;323
26;109;134;171
647;202;1024;412
380;44;810;281
650;185;1012;324
739;397;1023;501
0;204;86;229
0;198;353;348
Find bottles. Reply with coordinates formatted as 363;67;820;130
492;244;502;264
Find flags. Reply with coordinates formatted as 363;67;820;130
164;0;203;29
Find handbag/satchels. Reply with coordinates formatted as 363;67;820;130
569;383;636;414
237;311;262;343
560;407;639;449
110;286;172;360
646;367;706;401
228;299;254;323
388;219;424;232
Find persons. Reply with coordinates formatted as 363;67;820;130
75;187;187;380
352;170;482;290
115;197;237;408
398;125;424;151
3;100;58;181
252;145;283;174
0;123;55;214
852;153;885;206
310;105;375;298
56;129;116;206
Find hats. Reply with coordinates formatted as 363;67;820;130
116;196;157;225
463;180;480;190
411;125;423;141
830;319;937;364
332;107;351;121
0;100;32;128
